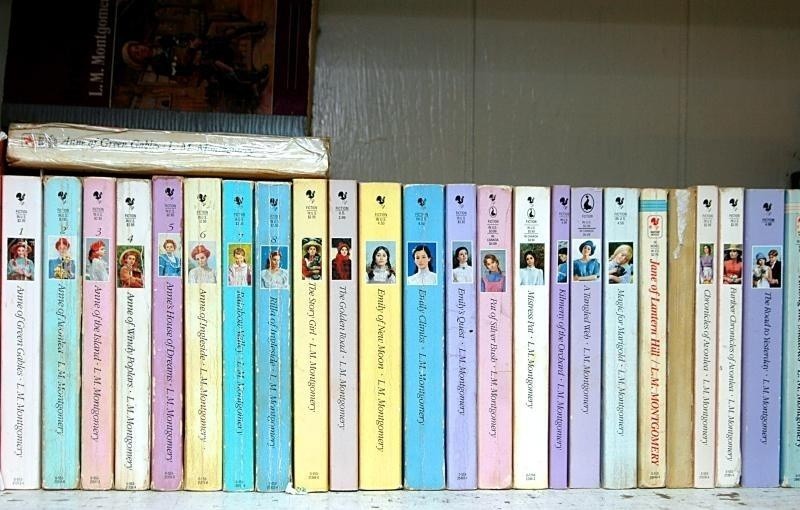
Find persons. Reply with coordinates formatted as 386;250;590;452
118;21;270;90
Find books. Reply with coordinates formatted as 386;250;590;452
0;172;800;492
0;0;318;136
4;119;334;182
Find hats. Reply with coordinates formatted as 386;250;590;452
11;242;31;253
338;242;349;249
579;241;595;256
119;249;141;266
756;252;767;264
724;244;742;255
303;240;322;252
